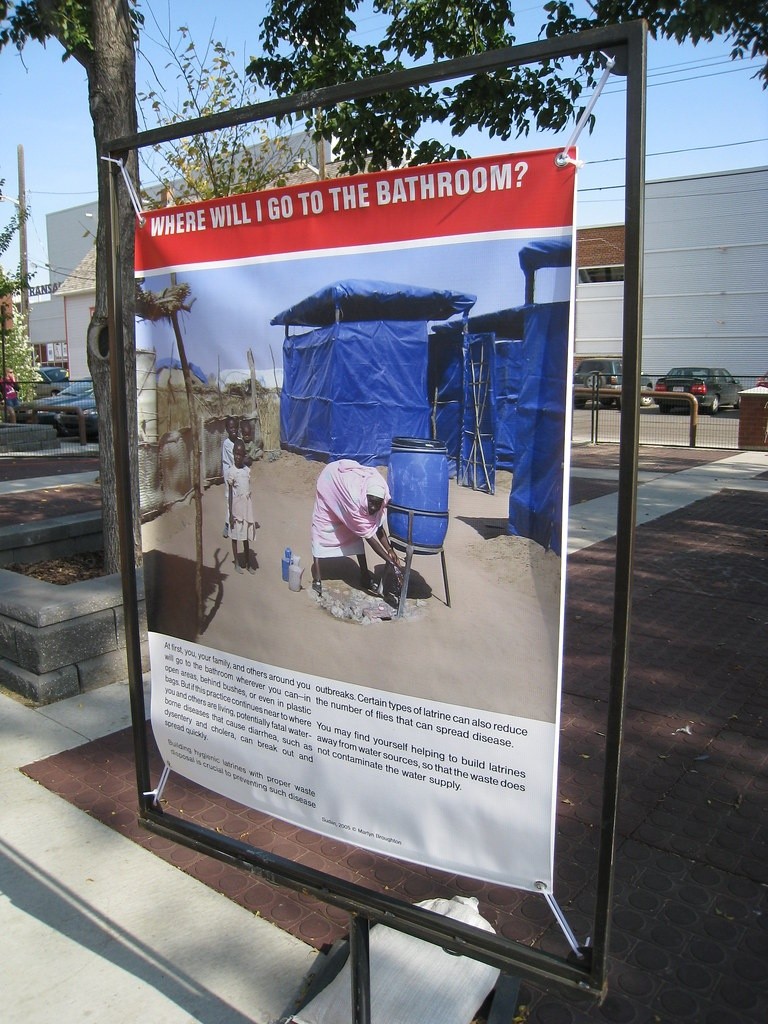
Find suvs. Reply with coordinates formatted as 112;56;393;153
573;358;654;410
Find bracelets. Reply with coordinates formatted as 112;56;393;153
388;548;392;553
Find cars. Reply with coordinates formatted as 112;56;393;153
12;377;100;442
30;366;69;396
652;366;743;415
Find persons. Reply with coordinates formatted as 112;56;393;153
223;418;256;574
311;459;403;595
756;370;768;388
0;368;20;423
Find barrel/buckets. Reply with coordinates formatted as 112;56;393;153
386;437;448;555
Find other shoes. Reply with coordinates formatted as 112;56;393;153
312;578;323;593
234;565;244;575
361;571;380;594
223;523;229;538
246;564;255;575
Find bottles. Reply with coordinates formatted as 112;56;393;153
289;556;302;592
282;548;292;582
